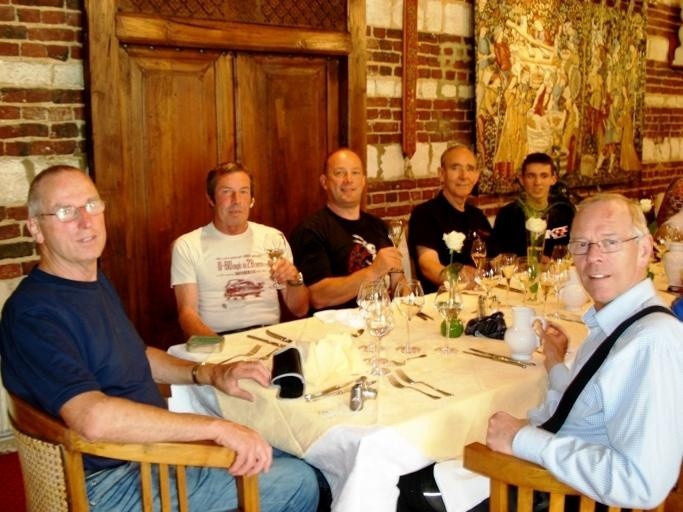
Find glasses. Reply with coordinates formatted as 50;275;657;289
567;236;642;255
30;198;106;222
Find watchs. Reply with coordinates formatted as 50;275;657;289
286;272;304;287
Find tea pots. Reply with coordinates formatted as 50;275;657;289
561;267;590;310
506;305;548;363
660;241;683;290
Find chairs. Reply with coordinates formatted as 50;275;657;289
464;442;666;511
6;393;260;512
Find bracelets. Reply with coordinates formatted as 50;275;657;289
189;359;211;386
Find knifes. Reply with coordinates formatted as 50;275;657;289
462;345;538;371
247;326;294;348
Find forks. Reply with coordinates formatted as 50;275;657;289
212;346;279;368
390;353;435;365
384;368;454;400
350;326;364;341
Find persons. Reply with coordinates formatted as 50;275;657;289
0;163;324;510
392;185;682;512
485;150;577;260
476;0;644;181
287;145;412;315
168;161;314;345
405;140;499;293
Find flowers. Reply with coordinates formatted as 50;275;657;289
640;198;659;280
438;230;466;305
509;192;570;300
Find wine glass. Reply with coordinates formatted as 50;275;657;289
356;220;569;377
